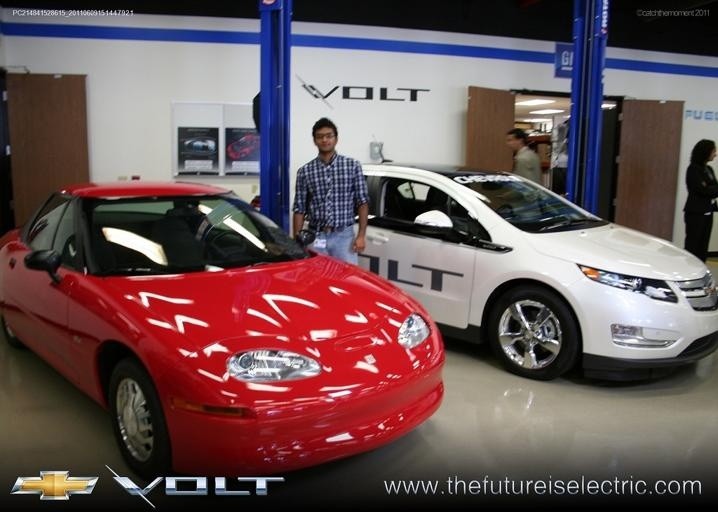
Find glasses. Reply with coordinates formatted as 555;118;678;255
314;134;336;139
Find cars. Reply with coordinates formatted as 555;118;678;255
253;162;718;382
1;181;444;485
227;135;261;160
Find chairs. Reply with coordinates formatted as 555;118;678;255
161;205;214;273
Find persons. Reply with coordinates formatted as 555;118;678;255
505;128;544;186
549;115;571;195
682;138;718;263
292;116;371;264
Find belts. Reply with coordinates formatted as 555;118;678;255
308;222;352;233
